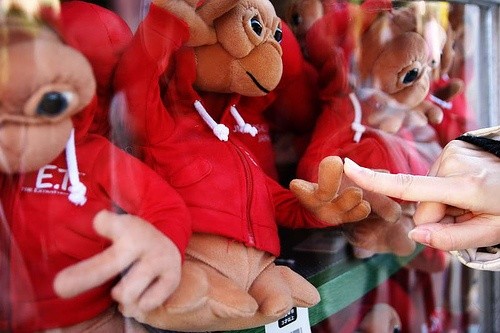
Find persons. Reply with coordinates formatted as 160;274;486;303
343;124;500;271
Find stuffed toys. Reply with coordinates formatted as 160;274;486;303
1;0;476;333
